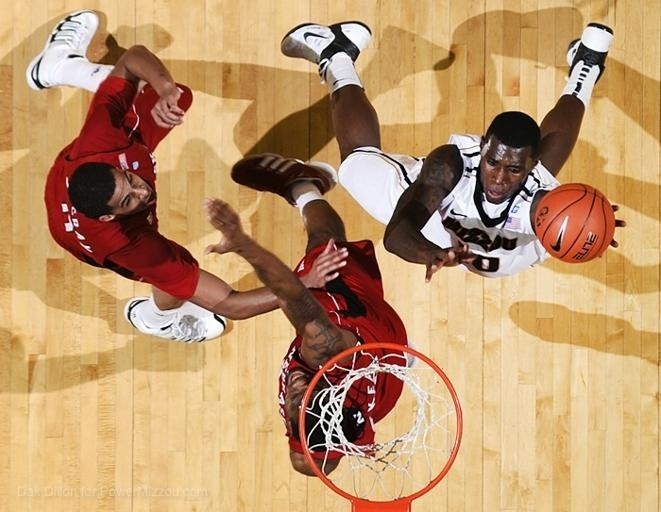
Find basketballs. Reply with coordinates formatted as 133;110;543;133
534;183;615;263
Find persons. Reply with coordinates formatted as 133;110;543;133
201;147;421;478
24;7;352;347
275;17;629;286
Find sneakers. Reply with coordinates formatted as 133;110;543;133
566;23;613;68
230;153;338;208
123;296;227;344
25;9;98;91
280;20;372;84
